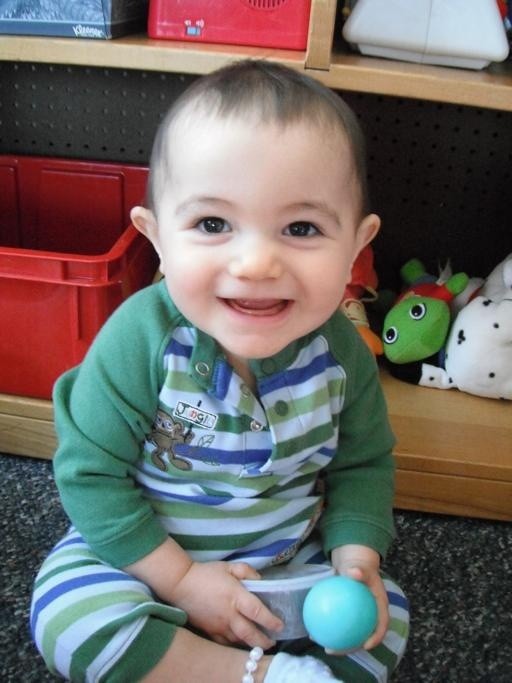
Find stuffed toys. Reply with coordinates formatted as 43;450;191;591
366;254;511;402
372;257;471;363
340;241;385;357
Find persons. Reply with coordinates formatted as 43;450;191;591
20;52;416;683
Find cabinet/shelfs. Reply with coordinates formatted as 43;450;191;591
0;0;511;525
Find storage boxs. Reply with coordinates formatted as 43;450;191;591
0;0;149;40
0;154;156;403
148;0;312;51
